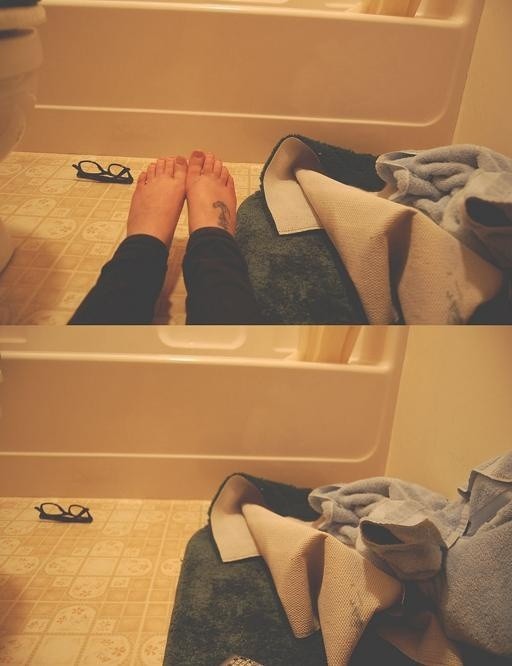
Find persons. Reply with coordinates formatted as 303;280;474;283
67;149;254;326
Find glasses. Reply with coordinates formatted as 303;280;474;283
71;158;134;184
34;501;93;525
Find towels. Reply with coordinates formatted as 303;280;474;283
370;144;512;270
309;451;512;666
